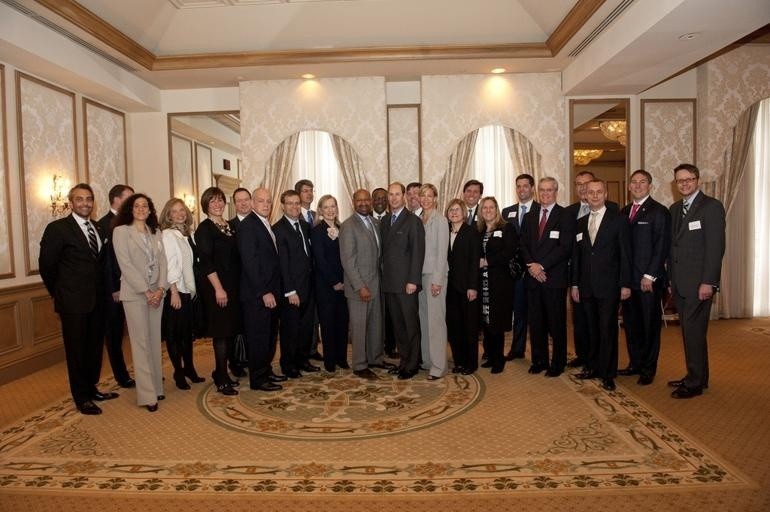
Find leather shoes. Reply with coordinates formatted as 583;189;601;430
528;355;703;399
252;347;525;392
75;377;135;415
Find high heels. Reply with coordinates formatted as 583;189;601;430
145;364;246;411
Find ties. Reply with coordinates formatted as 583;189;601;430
365;211;416;248
264;210;312;256
521;205;527;224
84;221;97;260
588;213;597;242
539;209;547;239
630;204;641;221
579;205;586;217
682;198;689;220
466;210;473;226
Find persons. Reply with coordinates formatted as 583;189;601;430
39;182;205;415
501;163;725;400
194;179;515;395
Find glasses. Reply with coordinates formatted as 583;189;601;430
675;178;697;184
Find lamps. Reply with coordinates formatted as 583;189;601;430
50;172;74;216
599;120;626;146
185;194;198;213
571;143;604;166
